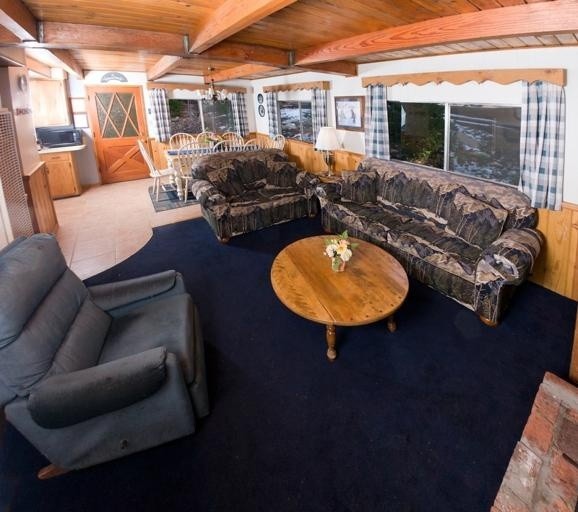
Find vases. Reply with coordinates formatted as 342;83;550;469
332;255;345;271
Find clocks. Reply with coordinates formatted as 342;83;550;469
257;93;263;103
258;105;265;117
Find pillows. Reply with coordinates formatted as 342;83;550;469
264;160;297;193
340;168;377;207
207;164;246;202
444;191;509;251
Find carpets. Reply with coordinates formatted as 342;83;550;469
0;216;578;511
148;182;200;213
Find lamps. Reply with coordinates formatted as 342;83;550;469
199;66;227;106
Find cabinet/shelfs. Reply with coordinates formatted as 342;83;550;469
22;160;60;237
39;135;87;200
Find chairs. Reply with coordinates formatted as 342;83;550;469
0;233;211;480
137;132;286;203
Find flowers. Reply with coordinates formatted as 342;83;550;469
323;228;361;262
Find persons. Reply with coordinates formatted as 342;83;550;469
349;109;355;123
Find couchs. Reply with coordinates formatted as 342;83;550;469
322;157;545;327
189;148;322;243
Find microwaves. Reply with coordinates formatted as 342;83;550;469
35;126;81;149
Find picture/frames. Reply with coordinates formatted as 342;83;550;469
334;95;364;133
